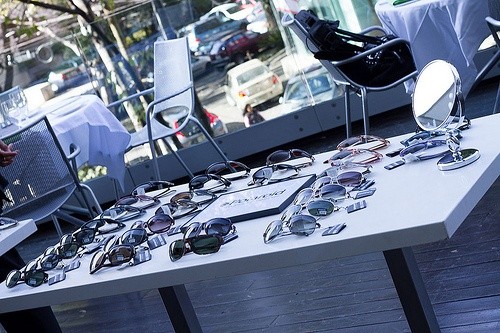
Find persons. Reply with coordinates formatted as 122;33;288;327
243;104;265;127
0;140;25;284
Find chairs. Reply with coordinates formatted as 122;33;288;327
104;38;238;201
280;8;419;143
485;14;500;114
0;115;104;239
0;218;39;257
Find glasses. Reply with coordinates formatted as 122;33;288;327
168;218;237;262
400;128;464;163
280;200;340;222
130;180;177;198
329;149;383;165
263;215;322;243
335;135;390;150
59;229;102;245
100;206;146;222
103;228;148;253
24;253;62;271
131;214;175;236
81;219;126;235
252;164;301;184
188;174;232;191
89;244;137;274
205;161;251;181
266;149;316;168
318;165;373;178
114;194;161;210
155;201;198;219
170;190;217;205
5;269;49;288
293;184;349;203
311;169;372;187
43;244;86;259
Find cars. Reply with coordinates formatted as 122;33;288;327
172;107;228;147
219;58;283;115
279;63;335;116
48;66;84;93
175;3;267;77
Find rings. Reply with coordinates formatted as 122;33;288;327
4;157;5;162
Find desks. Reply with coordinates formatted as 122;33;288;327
0;113;500;333
371;0;500;123
0;94;132;239
